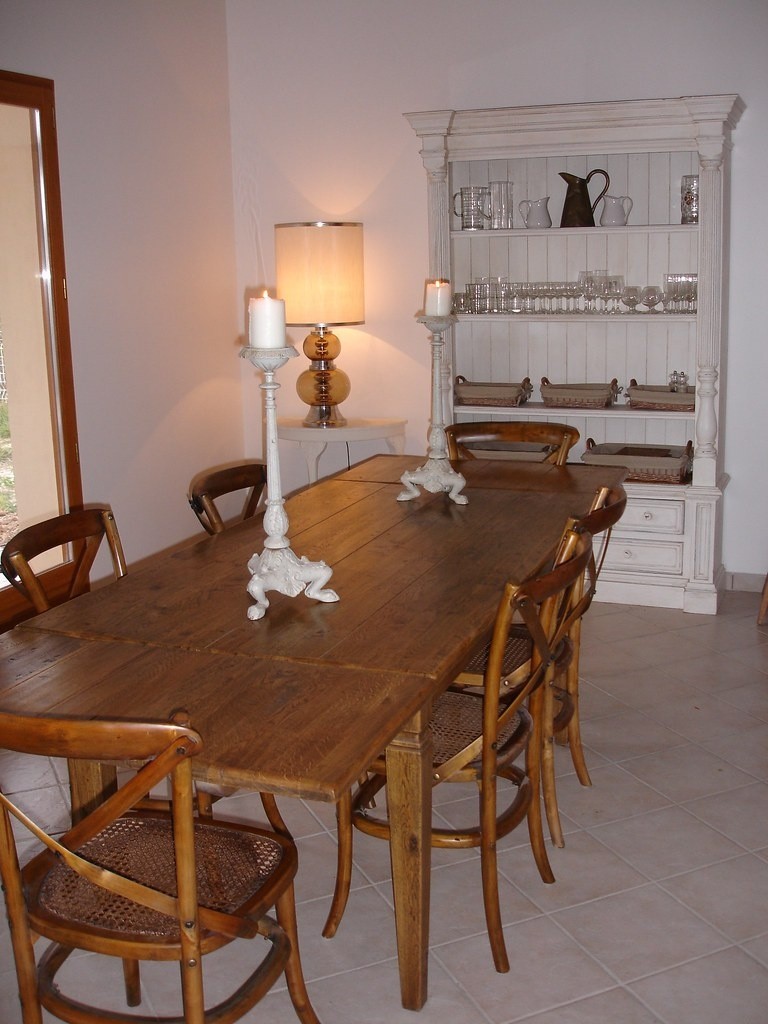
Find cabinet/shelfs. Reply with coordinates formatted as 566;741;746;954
402;93;747;614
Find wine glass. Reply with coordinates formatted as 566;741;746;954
506;270;698;315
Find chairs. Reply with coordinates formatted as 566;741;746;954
443;480;627;849
444;420;582;466
320;530;593;975
185;464;268;534
0;510;296;899
0;710;321;1024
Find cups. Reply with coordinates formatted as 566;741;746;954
680;174;700;226
453;186;487;231
477;181;514;230
452;276;507;315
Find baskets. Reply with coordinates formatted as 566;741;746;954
453;375;532;407
540;377;618;409
626;379;695;411
581;438;693;485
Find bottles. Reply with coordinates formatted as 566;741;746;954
668;370;680;392
677;372;688;392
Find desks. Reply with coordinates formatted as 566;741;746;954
0;453;627;1010
278;418;407;487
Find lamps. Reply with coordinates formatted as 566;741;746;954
274;222;365;429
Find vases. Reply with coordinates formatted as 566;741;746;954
681;175;699;224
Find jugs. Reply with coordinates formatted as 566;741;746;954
600;194;633;226
519;197;552;229
558;169;611;228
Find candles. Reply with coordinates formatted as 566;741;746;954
248;297;286;350
424;281;451;314
